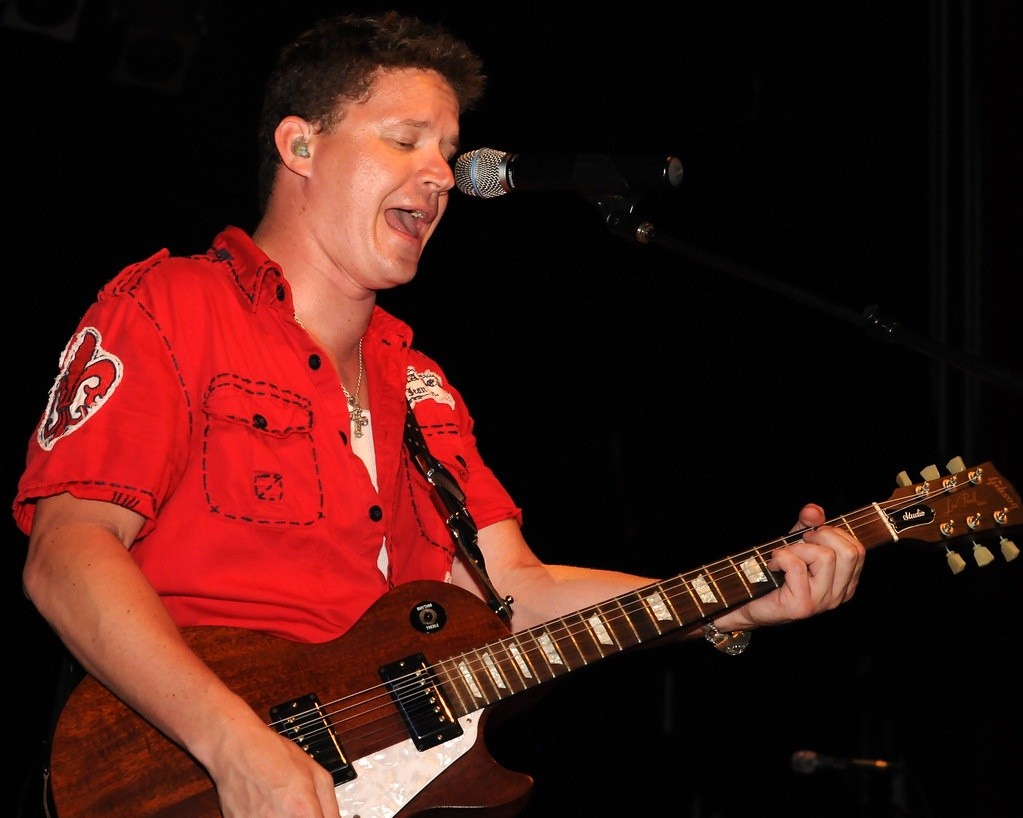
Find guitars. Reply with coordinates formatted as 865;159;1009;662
43;454;1023;818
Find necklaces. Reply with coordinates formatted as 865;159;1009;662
293;312;368;437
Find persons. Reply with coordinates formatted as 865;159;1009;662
0;11;865;818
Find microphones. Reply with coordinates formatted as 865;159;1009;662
792;750;892;774
454;146;685;197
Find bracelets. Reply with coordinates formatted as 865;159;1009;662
699;616;751;656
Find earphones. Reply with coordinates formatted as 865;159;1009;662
291;138;311;159
